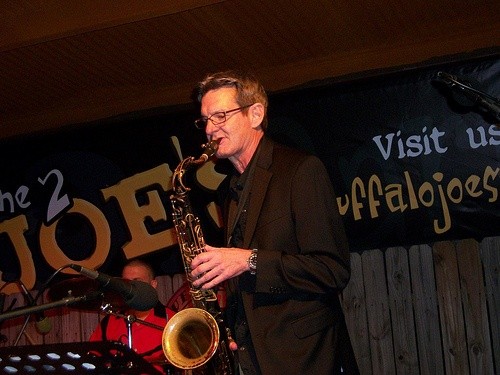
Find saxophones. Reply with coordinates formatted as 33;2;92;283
159;141;250;375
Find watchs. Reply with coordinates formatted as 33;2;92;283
247;249;258;275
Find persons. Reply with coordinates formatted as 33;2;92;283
190;70;360;375
88;261;178;375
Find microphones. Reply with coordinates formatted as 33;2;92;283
71;263;158;312
18;280;51;333
437;72;453;84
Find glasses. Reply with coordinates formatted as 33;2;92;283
194;103;254;130
